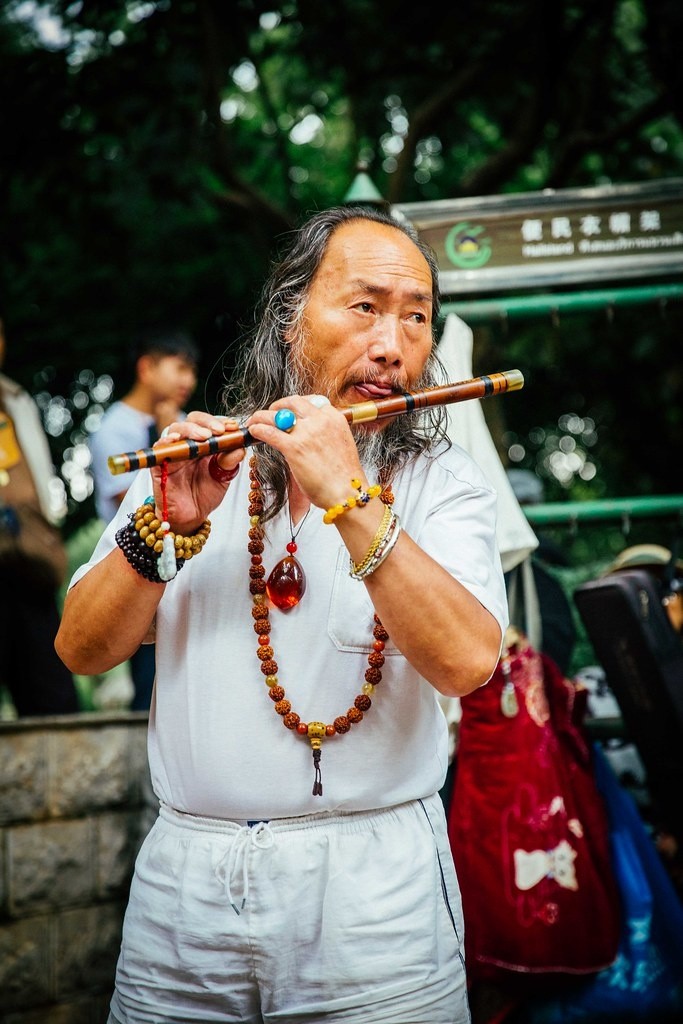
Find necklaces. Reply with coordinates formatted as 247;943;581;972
247;446;395;794
268;508;311;610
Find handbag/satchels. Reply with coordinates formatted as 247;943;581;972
448;557;622;973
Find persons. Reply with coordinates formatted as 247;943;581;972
0;329;82;718
91;332;195;704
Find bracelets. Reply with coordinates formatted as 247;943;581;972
208;452;240;482
353;493;402;579
115;496;212;583
323;480;383;522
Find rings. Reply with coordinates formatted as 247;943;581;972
52;208;511;1024
161;423;170;437
273;406;297;434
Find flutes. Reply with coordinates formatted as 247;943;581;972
108;366;523;476
